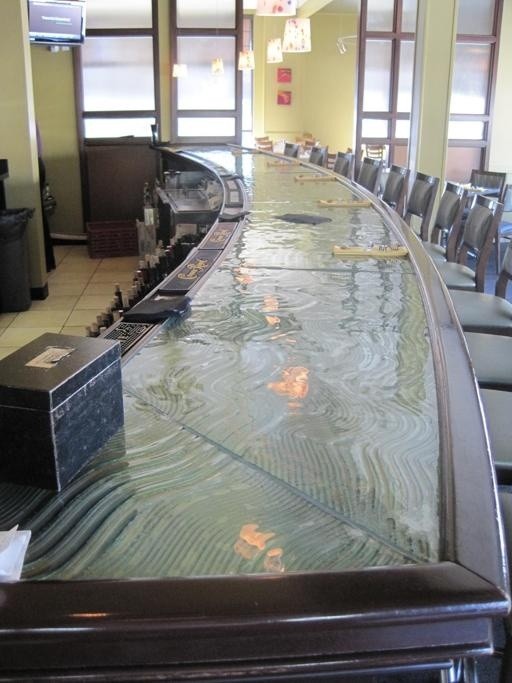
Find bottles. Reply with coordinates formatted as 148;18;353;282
85;226;207;338
151;124;160;144
142;170;181;225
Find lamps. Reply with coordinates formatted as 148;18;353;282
171;0;313;78
335;33;359;55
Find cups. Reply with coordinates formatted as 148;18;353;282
367;239;399;253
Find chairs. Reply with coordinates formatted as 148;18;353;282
252;133;512;683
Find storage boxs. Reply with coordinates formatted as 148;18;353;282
0;330;126;493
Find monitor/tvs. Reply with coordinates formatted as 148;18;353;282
28;0;85;45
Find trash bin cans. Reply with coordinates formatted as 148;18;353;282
0;208;31;312
